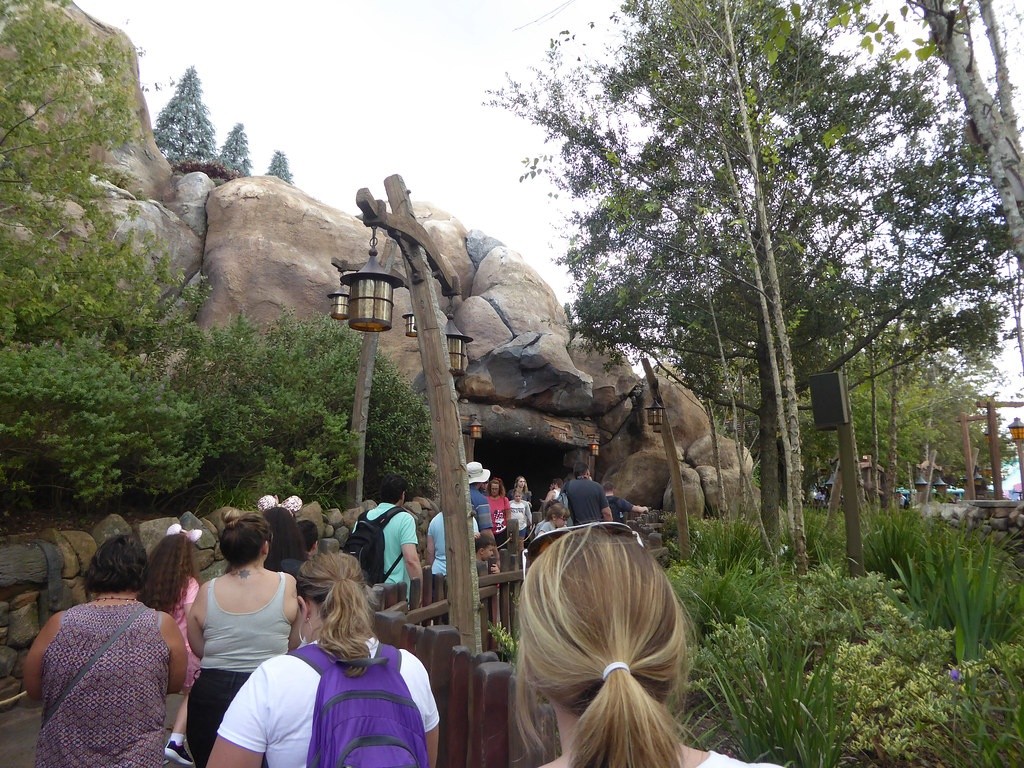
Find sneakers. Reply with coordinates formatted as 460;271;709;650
164;740;193;767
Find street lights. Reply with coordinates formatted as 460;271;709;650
1010;417;1024;498
328;192;484;656
648;396;691;565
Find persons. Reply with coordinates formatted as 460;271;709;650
896;491;906;508
519;528;786;768
23;534;189;768
187;507;299;768
205;550;440;768
262;461;649;624
135;532;200;766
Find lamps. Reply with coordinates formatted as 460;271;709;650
325;224;484;440
914;465;984;492
586;396;666;456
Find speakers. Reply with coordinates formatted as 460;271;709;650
809;373;848;428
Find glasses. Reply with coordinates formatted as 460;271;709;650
557;516;568;522
520;521;645;581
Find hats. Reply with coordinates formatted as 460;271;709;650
466;460;491;485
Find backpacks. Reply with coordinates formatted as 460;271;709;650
343;506;408;586
287;643;429;768
